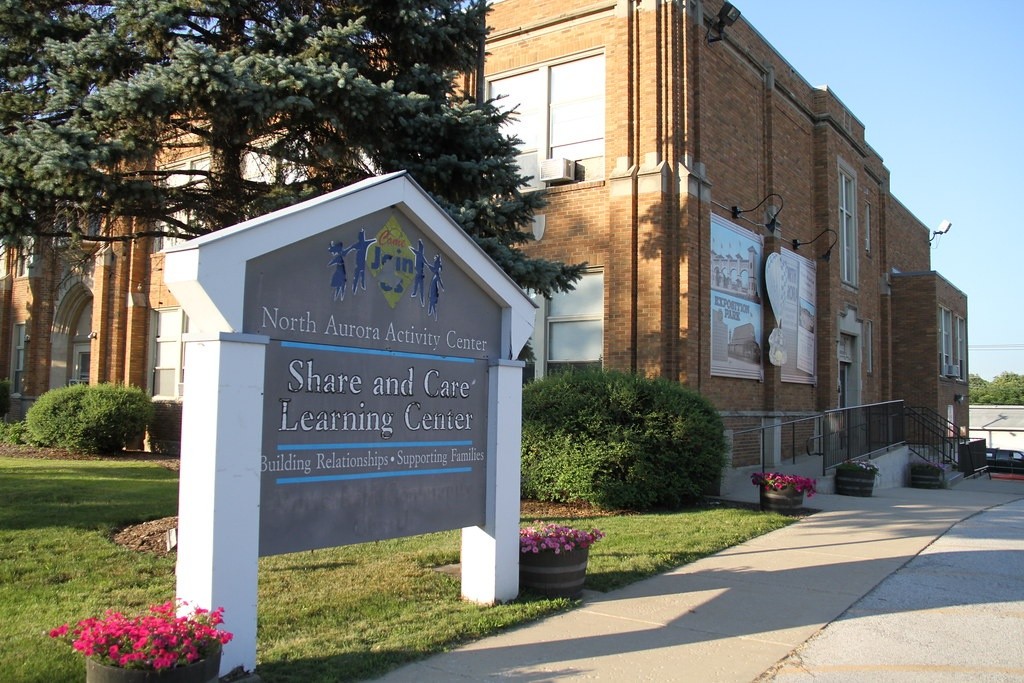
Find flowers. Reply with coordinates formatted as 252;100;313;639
519;519;606;554
909;460;945;472
49;596;234;672
751;471;817;497
838;459;880;475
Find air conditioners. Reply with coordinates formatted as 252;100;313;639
540;158;576;182
945;365;960;376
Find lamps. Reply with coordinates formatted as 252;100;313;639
793;228;838;263
706;2;741;43
732;194;784;233
954;393;963;403
929;219;952;242
88;331;97;340
24;334;31;343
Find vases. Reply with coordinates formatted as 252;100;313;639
837;471;874;496
520;550;589;596
760;486;804;514
85;657;220;683
911;470;939;489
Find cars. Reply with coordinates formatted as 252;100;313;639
986;448;1024;475
